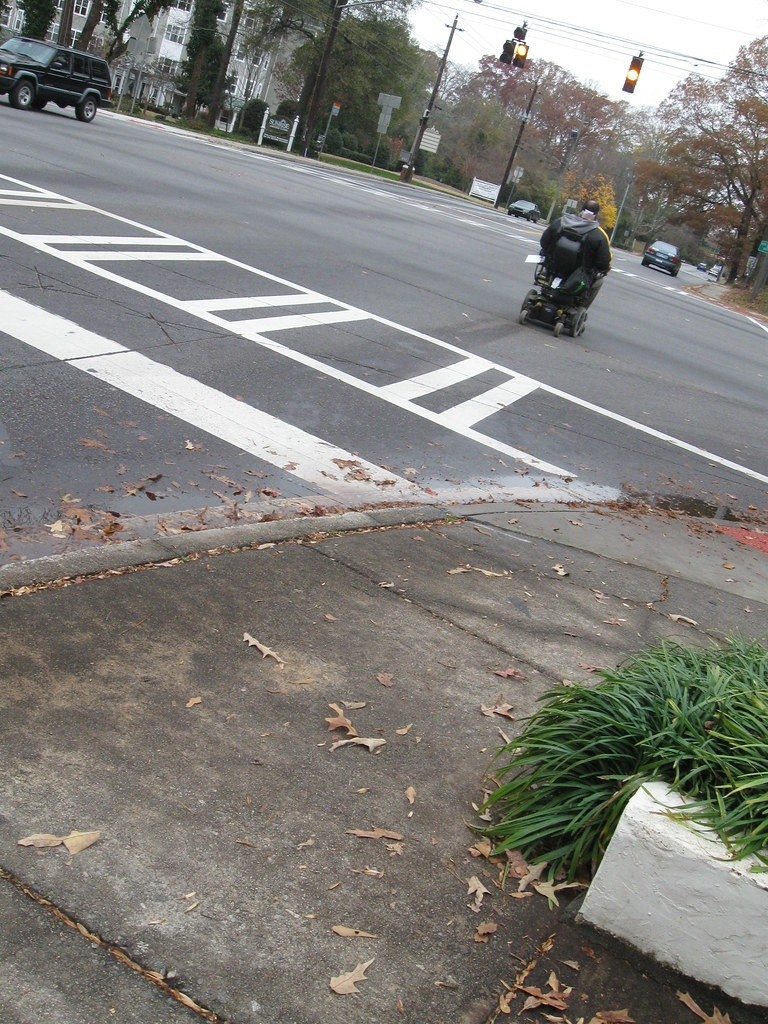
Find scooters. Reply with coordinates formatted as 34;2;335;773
518;246;606;338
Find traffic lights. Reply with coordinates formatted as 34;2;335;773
512;44;529;68
499;41;518;65
621;56;644;94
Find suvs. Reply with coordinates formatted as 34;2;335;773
0;37;115;123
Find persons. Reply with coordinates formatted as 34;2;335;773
540;199;612;316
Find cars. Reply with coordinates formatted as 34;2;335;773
708;264;722;277
640;240;683;277
697;263;708;272
508;200;541;224
